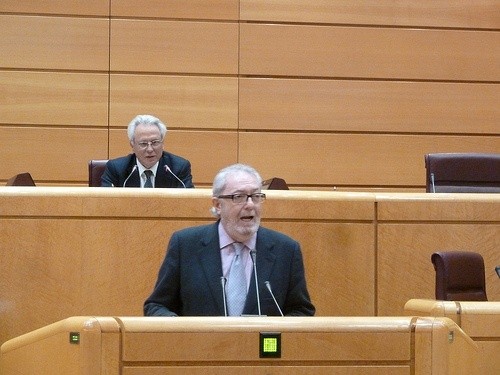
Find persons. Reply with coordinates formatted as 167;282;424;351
101;115;194;189
143;164;316;317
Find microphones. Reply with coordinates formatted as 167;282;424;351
123;165;138;187
430;173;435;193
250;249;261;317
264;280;284;317
219;277;228;317
163;165;186;188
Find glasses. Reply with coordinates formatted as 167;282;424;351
133;140;162;149
217;193;267;205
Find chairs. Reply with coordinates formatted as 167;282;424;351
431;250;488;301
425;153;500;193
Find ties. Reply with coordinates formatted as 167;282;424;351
143;170;153;188
224;242;247;316
